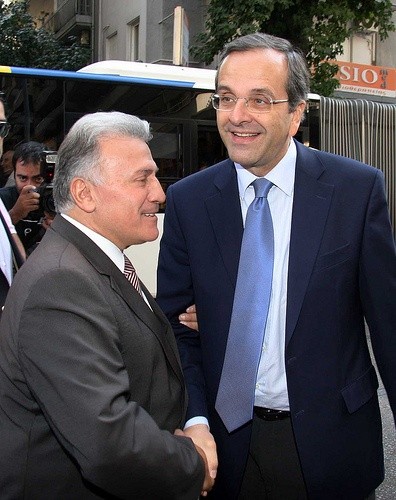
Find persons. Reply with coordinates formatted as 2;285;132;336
155;33;396;500
0;99;56;313
0;112;209;500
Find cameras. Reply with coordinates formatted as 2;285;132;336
29;150;57;217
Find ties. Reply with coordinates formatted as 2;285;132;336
124;254;143;303
214;179;275;433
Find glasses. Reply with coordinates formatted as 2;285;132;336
209;91;313;113
0;121;12;139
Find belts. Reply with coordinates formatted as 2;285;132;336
253;406;291;422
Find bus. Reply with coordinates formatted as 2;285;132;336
0;59;396;300
